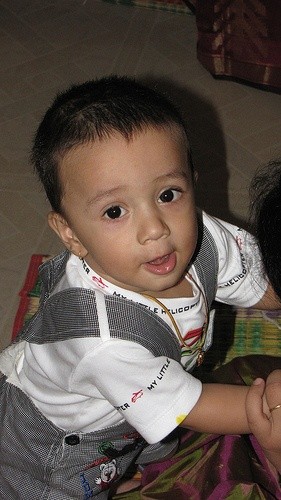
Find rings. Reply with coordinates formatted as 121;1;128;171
270;404;280;412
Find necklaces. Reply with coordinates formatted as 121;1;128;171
140;268;209;367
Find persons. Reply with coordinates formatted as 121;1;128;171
0;75;281;500
242;158;281;476
